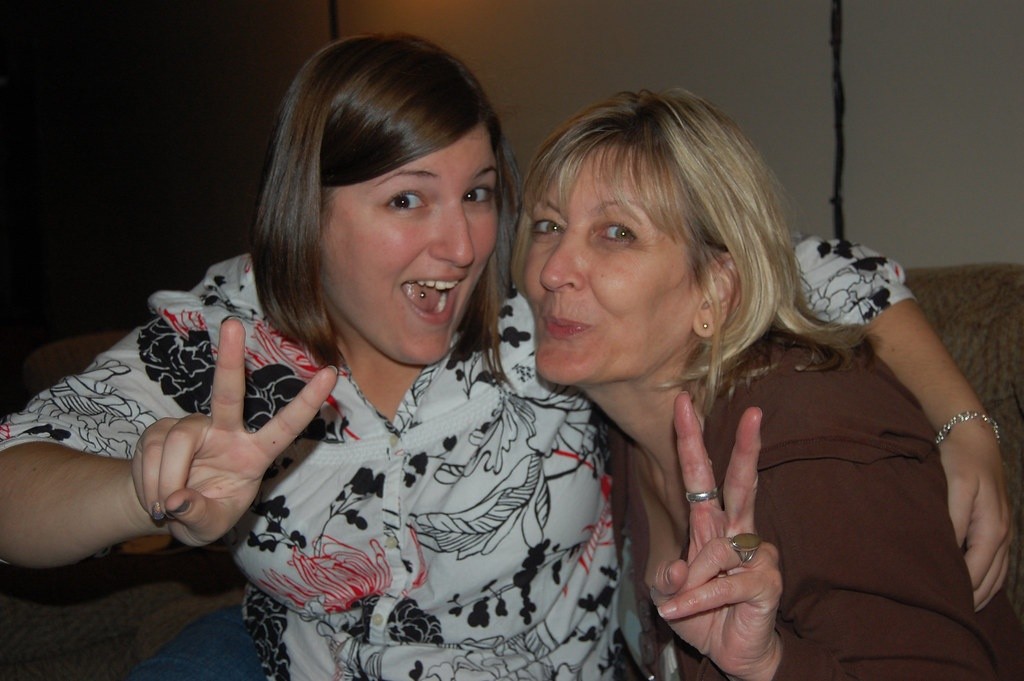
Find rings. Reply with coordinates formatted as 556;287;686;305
685;488;718;502
730;533;763;563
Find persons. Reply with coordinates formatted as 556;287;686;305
0;33;1008;680
513;86;986;679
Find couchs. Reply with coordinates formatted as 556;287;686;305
0;263;1023;681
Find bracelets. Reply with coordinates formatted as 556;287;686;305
935;411;1000;444
